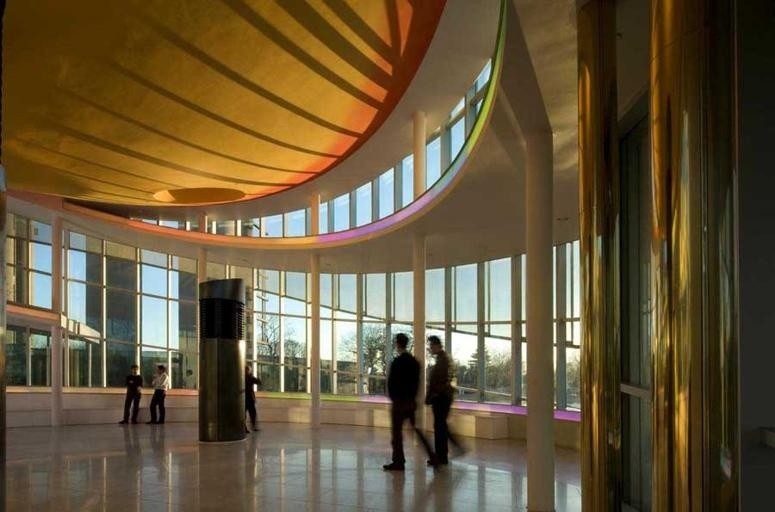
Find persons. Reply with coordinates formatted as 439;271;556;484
119;365;143;424
146;366;168;424
183;369;196;389
245;366;261;433
383;333;465;471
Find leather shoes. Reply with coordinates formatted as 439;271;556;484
246;428;261;433
383;462;405;471
118;420;138;425
145;419;165;424
427;455;449;466
